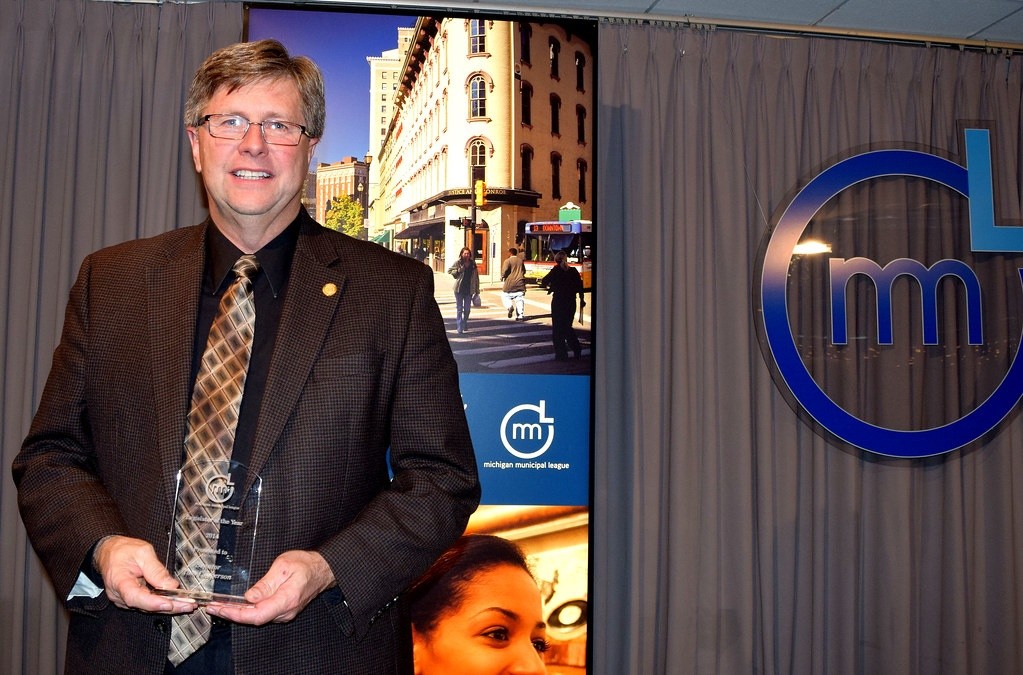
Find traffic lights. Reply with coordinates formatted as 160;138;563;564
449;217;462;230
474;180;487;207
460;216;474;227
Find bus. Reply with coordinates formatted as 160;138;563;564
520;217;592;292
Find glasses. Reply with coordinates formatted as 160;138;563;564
191;114;315;146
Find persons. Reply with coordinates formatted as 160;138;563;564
10;37;484;675
500;247;529;323
445;247;480;333
541;253;587;360
403;527;549;675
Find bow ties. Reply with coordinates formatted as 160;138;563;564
165;254;261;668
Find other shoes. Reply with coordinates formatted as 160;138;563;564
464;326;467;331
555;354;568;361
516;317;524;322
574;346;581;359
458;329;463;334
508;307;514;318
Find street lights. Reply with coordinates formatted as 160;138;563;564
363;150;373;240
358;183;364;241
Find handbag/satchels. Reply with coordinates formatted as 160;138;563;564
473;293;481;307
